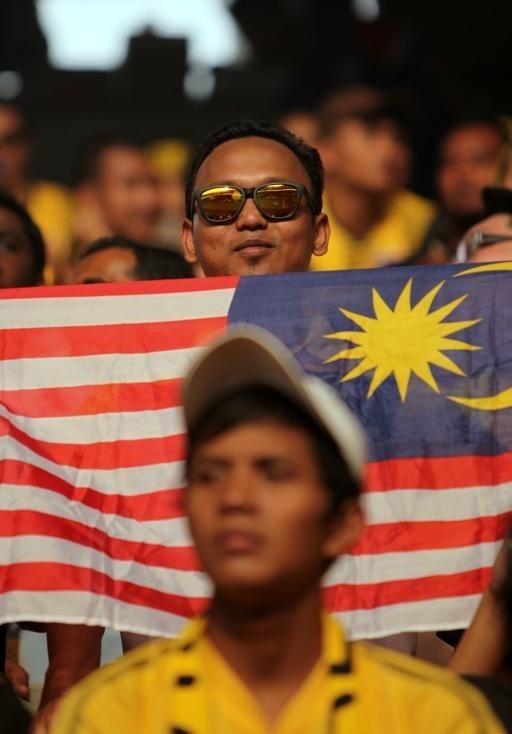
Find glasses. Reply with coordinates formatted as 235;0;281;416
185;178;319;225
455;232;512;262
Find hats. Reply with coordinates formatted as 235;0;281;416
180;319;367;490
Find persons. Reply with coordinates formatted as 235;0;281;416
0;75;511;734
